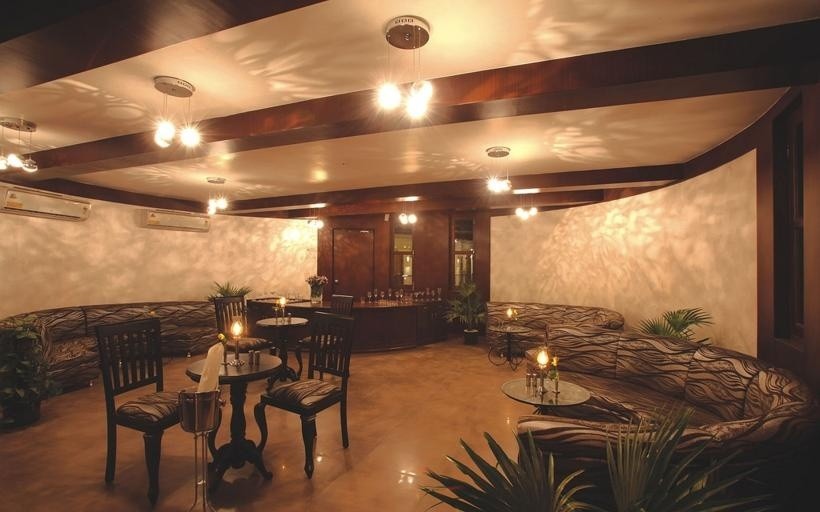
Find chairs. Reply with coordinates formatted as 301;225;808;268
95;318;222;499
214;295;276;355
254;325;355;478
296;294;354;379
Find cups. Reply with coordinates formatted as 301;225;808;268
361;297;365;304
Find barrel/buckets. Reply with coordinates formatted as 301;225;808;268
180;387;221;431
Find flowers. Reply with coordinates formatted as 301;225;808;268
304;275;328;296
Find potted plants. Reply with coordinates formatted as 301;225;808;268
0;315;62;429
441;281;485;344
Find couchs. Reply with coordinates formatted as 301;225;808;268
0;301;219;396
517;323;819;508
486;302;624;354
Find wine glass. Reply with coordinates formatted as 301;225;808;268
413;288;443;301
366;287;404;303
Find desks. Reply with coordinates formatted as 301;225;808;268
256;316;310;379
186;354;282;500
487;324;532;371
500;377;591;415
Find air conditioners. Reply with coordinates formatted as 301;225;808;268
141;209;211;232
0;186;91;222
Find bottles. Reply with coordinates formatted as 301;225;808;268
526;373;530;386
532;387;537;394
526;386;530;394
248;350;261;365
533;373;537;387
287;313;292;321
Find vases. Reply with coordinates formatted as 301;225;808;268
309;290;322;304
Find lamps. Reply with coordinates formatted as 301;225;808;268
396;195;420;224
375;14;435;121
486;145;512;192
152;75;203;150
206;177;229;217
0;117;37;173
